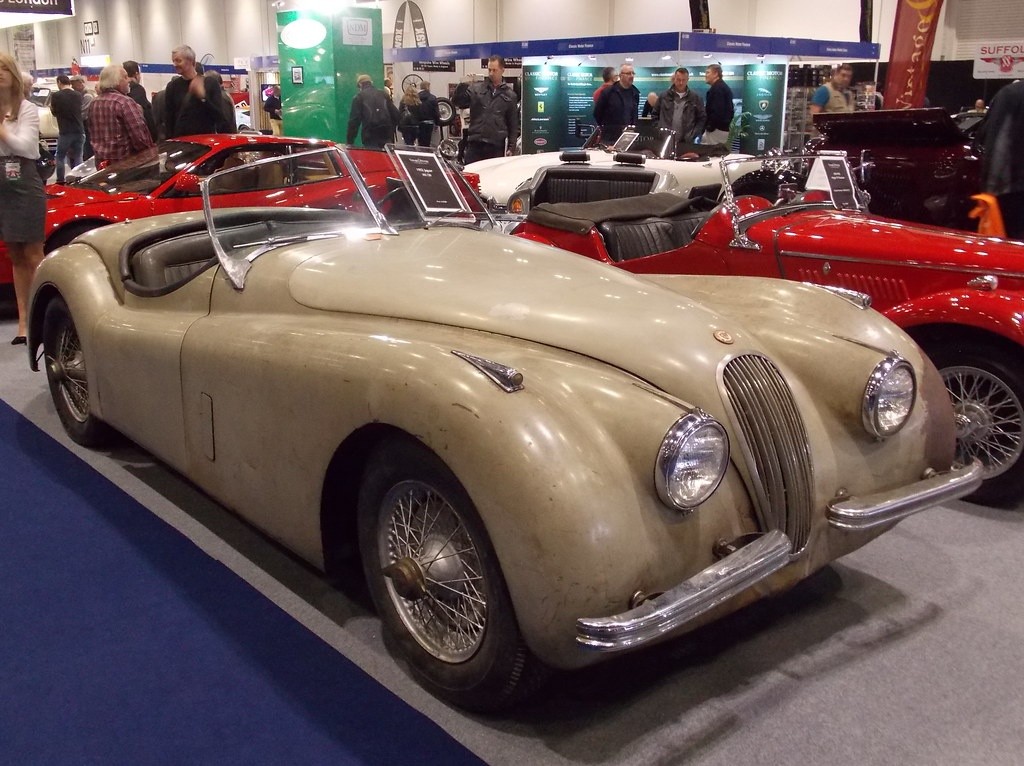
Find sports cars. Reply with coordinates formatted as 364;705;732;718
27;142;983;723
0;129;484;285
461;107;1024;512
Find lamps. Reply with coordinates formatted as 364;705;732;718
544;55;553;65
704;53;722;65
578;55;596;66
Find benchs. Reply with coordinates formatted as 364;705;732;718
136;222;337;293
595;208;710;263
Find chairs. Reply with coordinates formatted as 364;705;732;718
258;161;292;190
216;157;260;193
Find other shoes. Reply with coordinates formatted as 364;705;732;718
11;333;26;345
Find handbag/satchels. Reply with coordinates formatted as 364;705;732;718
971;194;1007;240
398;103;412;124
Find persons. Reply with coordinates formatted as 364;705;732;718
594;63;639;143
70;77;94;161
642;92;657;117
452;55;517;165
701;64;734;145
123;61;152;135
809;64;854;138
977;80;1024;240
385;79;392;100
152;44;237;137
87;63;153;169
593;67;618;103
21;72;33;100
51;76;85;182
398;81;441;145
654;68;707;144
0;51;47;344
968;99;988;113
346;74;400;147
264;86;282;135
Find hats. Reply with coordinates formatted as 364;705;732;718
69;75;85;83
356;74;372;87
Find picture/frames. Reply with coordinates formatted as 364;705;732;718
384;143;477;224
291;66;303;84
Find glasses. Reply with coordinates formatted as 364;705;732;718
621;71;635;77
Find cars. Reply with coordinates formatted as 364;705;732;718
800;107;988;243
